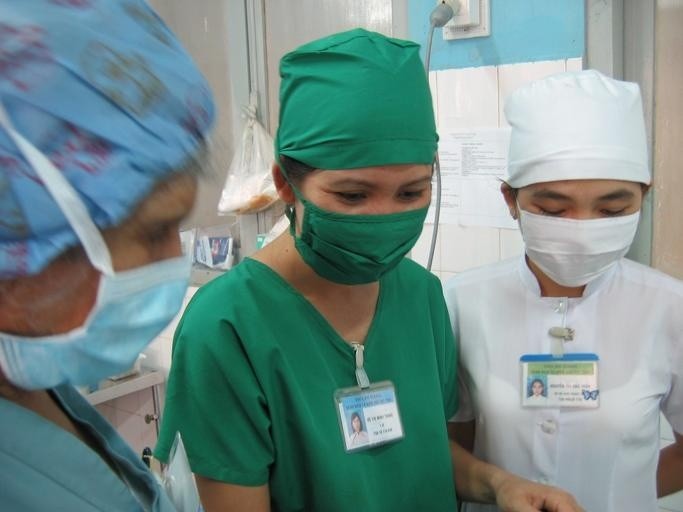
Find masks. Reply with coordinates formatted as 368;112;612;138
513;199;641;288
0;113;192;391
284;172;429;284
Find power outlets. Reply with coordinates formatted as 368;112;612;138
437;0;491;42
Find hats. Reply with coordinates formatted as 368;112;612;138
506;70;652;189
275;28;440;169
1;0;215;278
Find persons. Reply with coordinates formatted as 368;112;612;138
0;0;220;511
347;411;365;445
441;68;682;511
152;24;590;512
527;376;543;402
